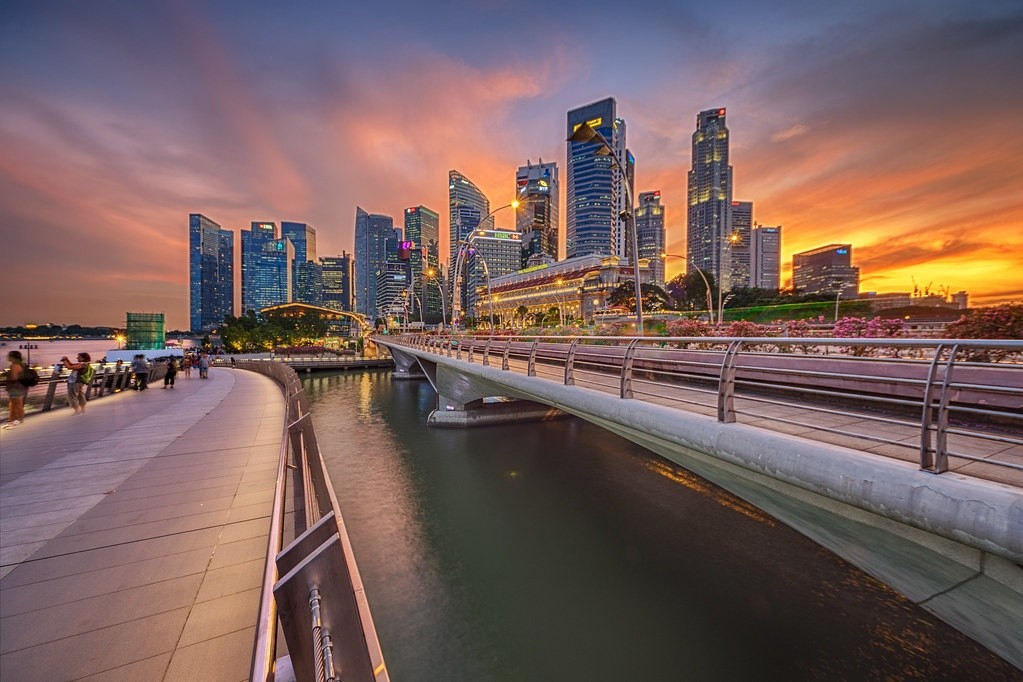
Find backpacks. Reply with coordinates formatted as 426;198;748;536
13;362;38;387
78;362;95;383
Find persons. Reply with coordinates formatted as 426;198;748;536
231;357;236;369
182;355;193;379
60;352;96;418
2;350;32;430
197;351;211;379
159;355;177;389
128;354;149;392
133;353;152;390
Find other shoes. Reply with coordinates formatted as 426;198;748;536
5;420;22;426
200;375;208;379
71;411;88;417
184;375;190;380
160;386;174;389
133;386;150;391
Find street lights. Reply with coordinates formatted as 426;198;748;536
116;336;124;350
452;201;520;332
565;122;644;337
718;235;739;325
458;238;494;333
427;269;447;330
661;255;714;326
